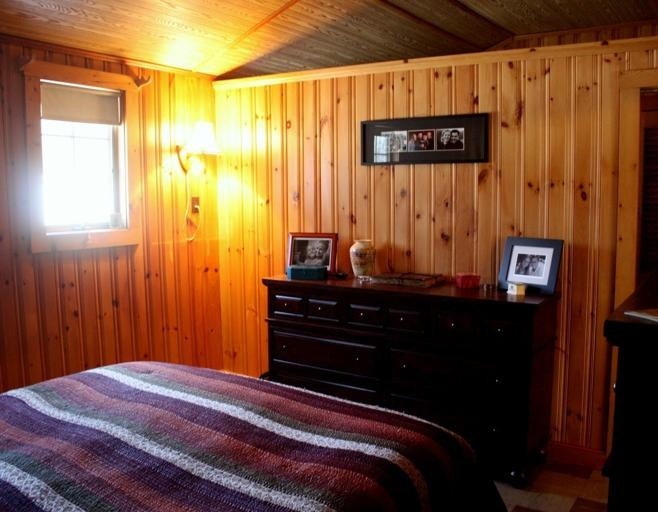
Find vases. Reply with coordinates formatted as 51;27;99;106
348;235;378;281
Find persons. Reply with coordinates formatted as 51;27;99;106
293;241;329;265
381;131;463;150
515;254;542;276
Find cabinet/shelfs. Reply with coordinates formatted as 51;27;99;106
601;290;658;512
261;271;560;484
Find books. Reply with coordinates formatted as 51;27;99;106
372;272;441;289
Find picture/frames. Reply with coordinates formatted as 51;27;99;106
494;235;563;295
359;113;489;165
285;232;338;275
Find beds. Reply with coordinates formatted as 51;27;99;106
0;360;505;512
